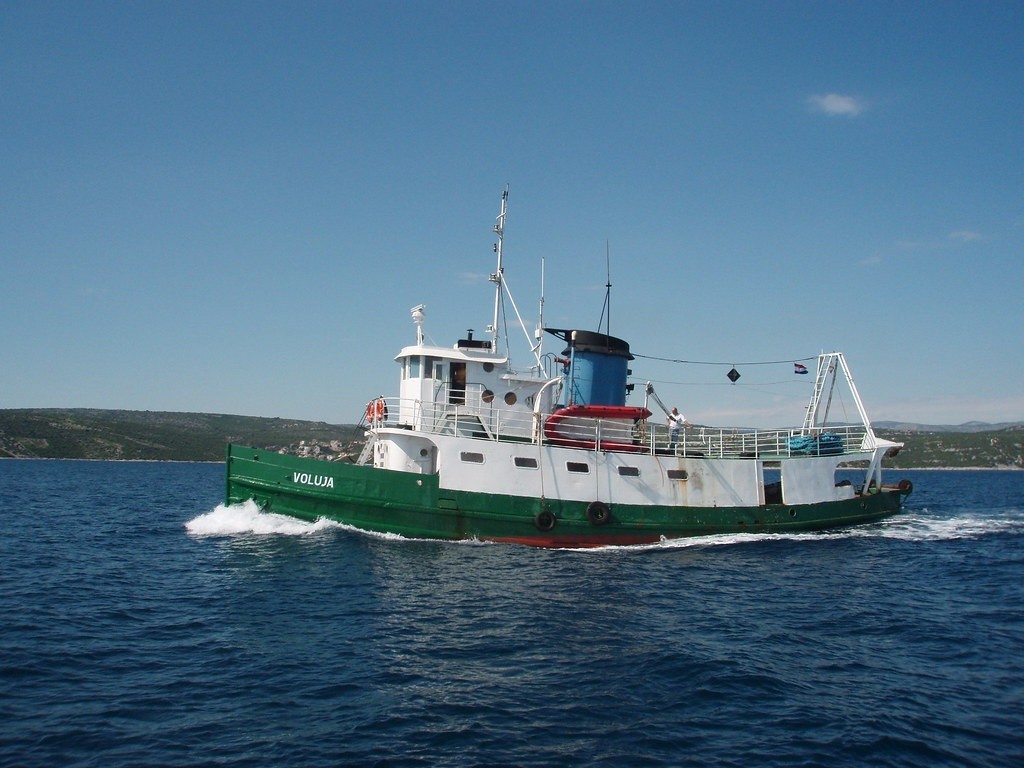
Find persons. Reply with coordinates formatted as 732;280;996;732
454;364;466;403
427;366;439;379
666;407;693;455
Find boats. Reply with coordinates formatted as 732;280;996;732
223;181;914;552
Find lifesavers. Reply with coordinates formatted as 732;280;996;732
586;501;613;526
376;401;384;422
367;402;375;423
533;511;556;533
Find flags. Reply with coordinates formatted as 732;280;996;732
794;363;808;374
